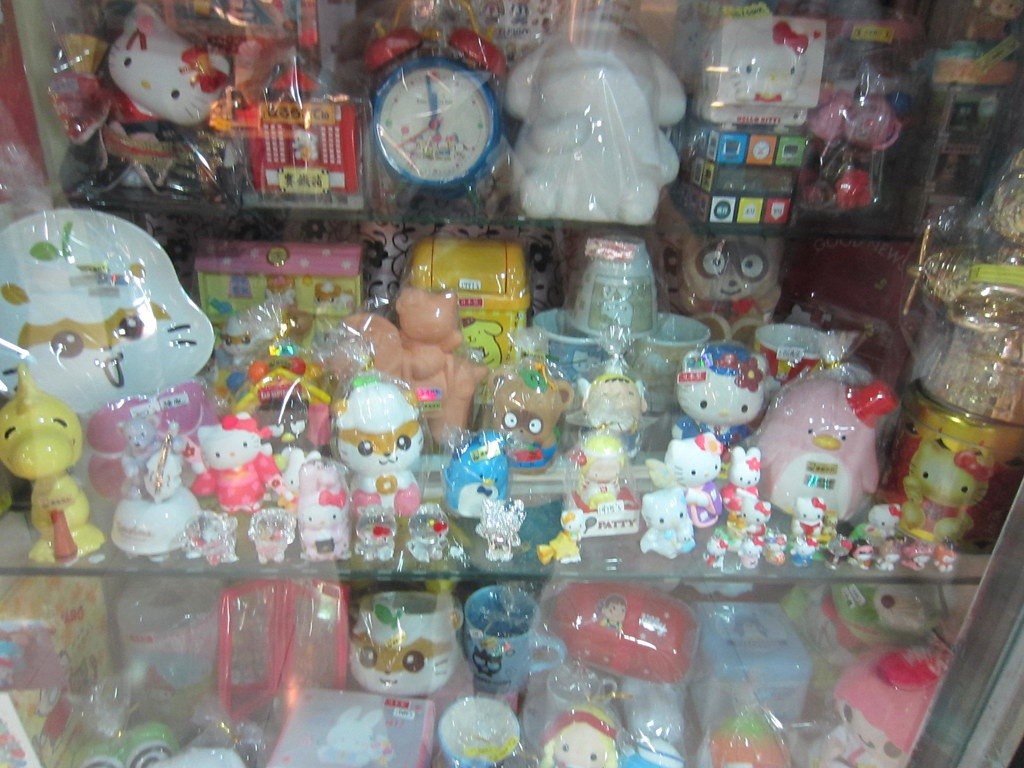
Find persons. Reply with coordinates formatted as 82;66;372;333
543;705;619;768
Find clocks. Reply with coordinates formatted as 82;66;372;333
364;0;507;208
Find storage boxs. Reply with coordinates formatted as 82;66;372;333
268;692;436;768
690;597;812;730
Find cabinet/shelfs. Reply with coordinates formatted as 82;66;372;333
0;197;1024;768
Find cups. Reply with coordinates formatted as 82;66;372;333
350;591;465;696
439;697;519;768
628;313;712;414
749;324;821;431
549;666;617;718
532;309;610;385
465;585;563;694
569;235;657;337
